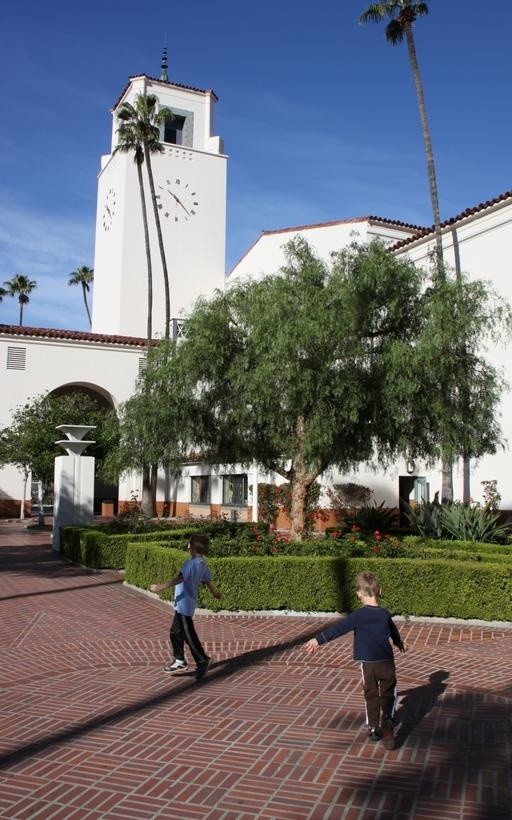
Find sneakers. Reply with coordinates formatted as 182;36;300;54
164;659;188;673
368;727;381;740
195;658;212;678
381;719;397;750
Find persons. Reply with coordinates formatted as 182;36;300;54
148;533;223;682
303;573;411;752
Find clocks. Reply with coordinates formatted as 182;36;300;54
100;189;117;232
155;179;198;223
